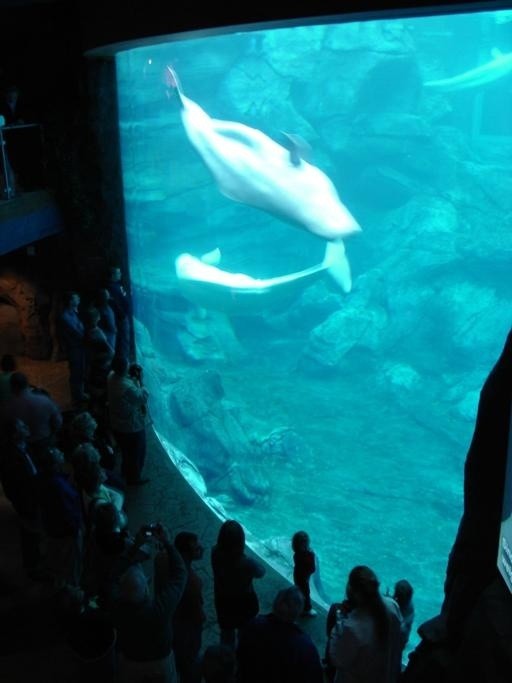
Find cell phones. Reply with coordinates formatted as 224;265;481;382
143;529;155;537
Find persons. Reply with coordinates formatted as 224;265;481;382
108;521;416;683
0;263;148;682
